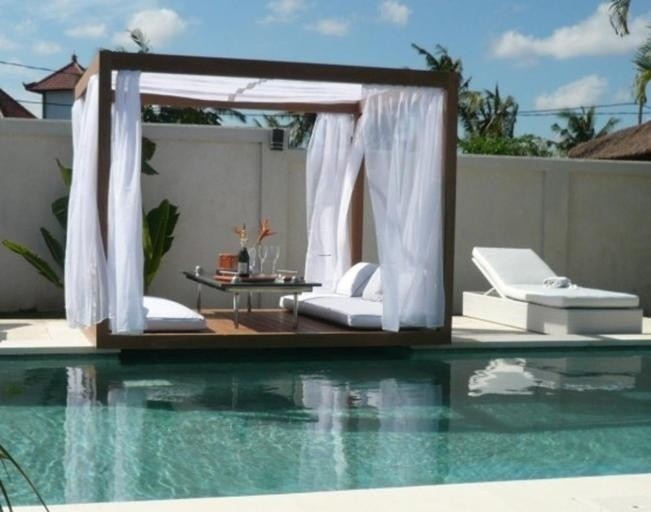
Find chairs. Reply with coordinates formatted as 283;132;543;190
462;246;643;335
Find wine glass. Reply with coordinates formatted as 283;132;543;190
257;244;280;276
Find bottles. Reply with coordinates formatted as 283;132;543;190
237;239;250;278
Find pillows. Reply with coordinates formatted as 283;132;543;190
337;262;378;297
362;266;383;302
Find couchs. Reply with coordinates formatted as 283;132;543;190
279;271;427;328
142;296;206;332
